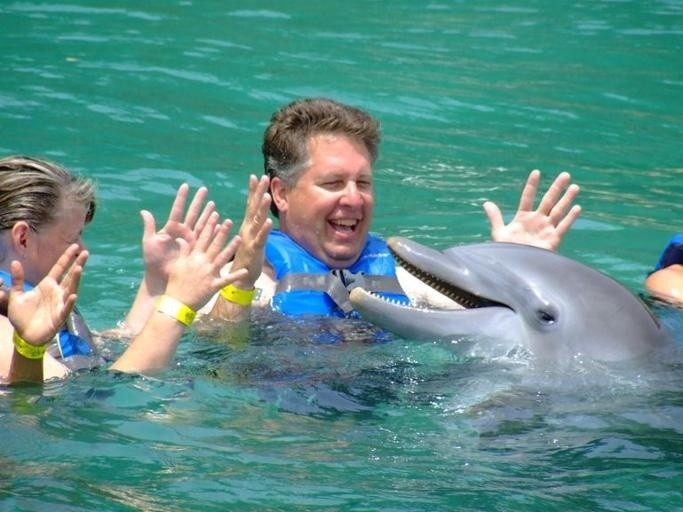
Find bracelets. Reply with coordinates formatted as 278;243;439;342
11;329;49;360
155;294;196;328
220;282;255;307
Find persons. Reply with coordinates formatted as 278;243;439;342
642;236;682;308
4;243;90;388
197;95;583;418
0;151;248;383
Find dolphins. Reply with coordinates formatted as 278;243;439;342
347;234;673;417
